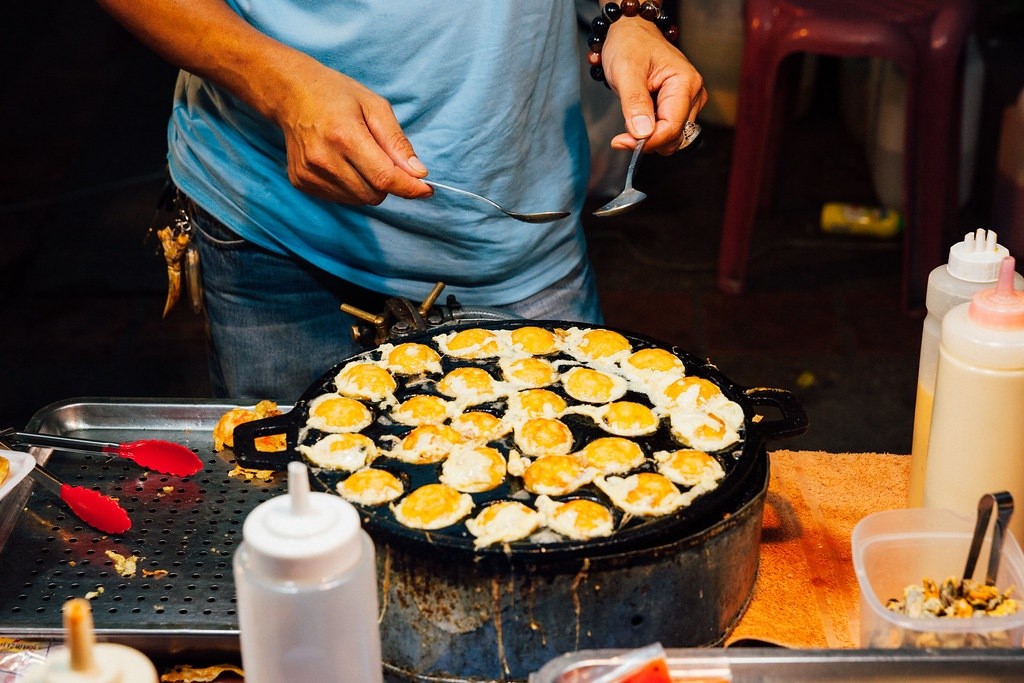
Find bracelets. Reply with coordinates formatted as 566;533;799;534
587;0;679;91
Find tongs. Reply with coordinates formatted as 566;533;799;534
3;432;204;533
959;490;1014;587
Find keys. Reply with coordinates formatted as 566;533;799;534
183;245;204;315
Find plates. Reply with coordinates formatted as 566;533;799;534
0;449;36;499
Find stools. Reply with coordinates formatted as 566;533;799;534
715;0;976;320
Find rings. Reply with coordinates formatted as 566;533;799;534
678;121;701;150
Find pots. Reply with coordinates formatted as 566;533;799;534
233;320;810;556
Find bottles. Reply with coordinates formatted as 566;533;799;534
909;228;1023;509
233;461;382;683
923;257;1024;549
822;203;903;239
20;598;159;683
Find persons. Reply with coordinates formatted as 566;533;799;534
100;0;708;400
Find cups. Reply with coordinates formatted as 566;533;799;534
851;507;1023;649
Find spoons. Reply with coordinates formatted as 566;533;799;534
418;178;571;223
593;138;647;217
